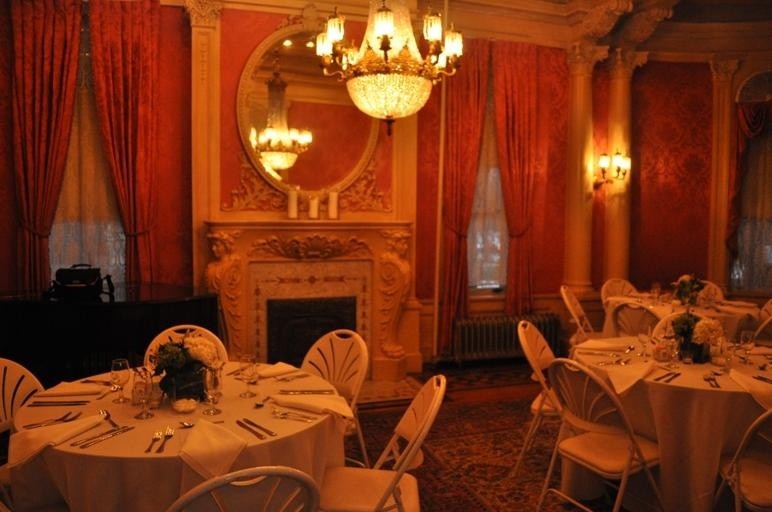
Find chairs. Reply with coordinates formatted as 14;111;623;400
0;354;45;435
319;372;448;512
298;329;370;467
142;324;230;362
163;465;318;511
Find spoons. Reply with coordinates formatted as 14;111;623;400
255;396;272;407
178;419;225;428
99;409;120;429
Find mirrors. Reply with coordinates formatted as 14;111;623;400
236;5;381;197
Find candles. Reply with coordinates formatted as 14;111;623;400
288;188;339;219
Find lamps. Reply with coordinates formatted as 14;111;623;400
249;56;313;173
594;146;634;189
315;0;463;136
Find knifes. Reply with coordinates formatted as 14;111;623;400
235;420;266;442
243;411;283;436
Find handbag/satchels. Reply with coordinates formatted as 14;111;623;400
56;264;102;297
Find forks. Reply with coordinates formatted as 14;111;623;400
154;424;175;453
144;431;163;452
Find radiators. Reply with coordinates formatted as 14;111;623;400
454;308;560;360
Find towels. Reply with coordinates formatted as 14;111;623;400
271;390;355;421
34;378;101;399
7;411;107;470
256;359;305;382
176;417;247;481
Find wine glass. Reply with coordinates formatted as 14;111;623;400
202;367;222;416
633;282;739;310
579;321;771;389
133;371;155;419
110;359;131;404
239;353;257;399
